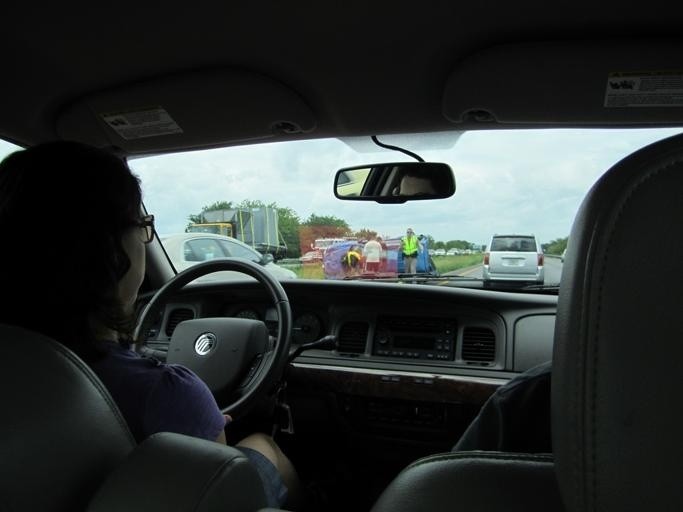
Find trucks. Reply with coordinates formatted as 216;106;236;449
190;207;288;264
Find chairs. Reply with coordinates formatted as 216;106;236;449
369;131;682;512
1;323;267;511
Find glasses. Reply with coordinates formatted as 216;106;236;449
134;215;154;243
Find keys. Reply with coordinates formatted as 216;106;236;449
270;379;293;434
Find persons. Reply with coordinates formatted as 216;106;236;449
452;359;553;452
399;171;443;197
346;228;423;284
0;149;301;504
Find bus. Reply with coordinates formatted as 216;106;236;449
310;238;345;251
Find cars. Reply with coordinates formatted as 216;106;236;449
158;232;297;286
428;247;472;257
299;251;323;263
482;232;545;288
